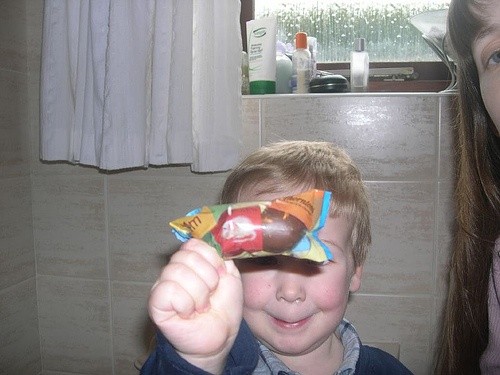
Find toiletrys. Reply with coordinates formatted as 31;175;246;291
292;33;311;94
350;38;370;92
257;52;293;94
294;36;318;93
245;16;277;95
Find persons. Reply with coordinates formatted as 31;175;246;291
430;0;500;375
138;135;414;375
